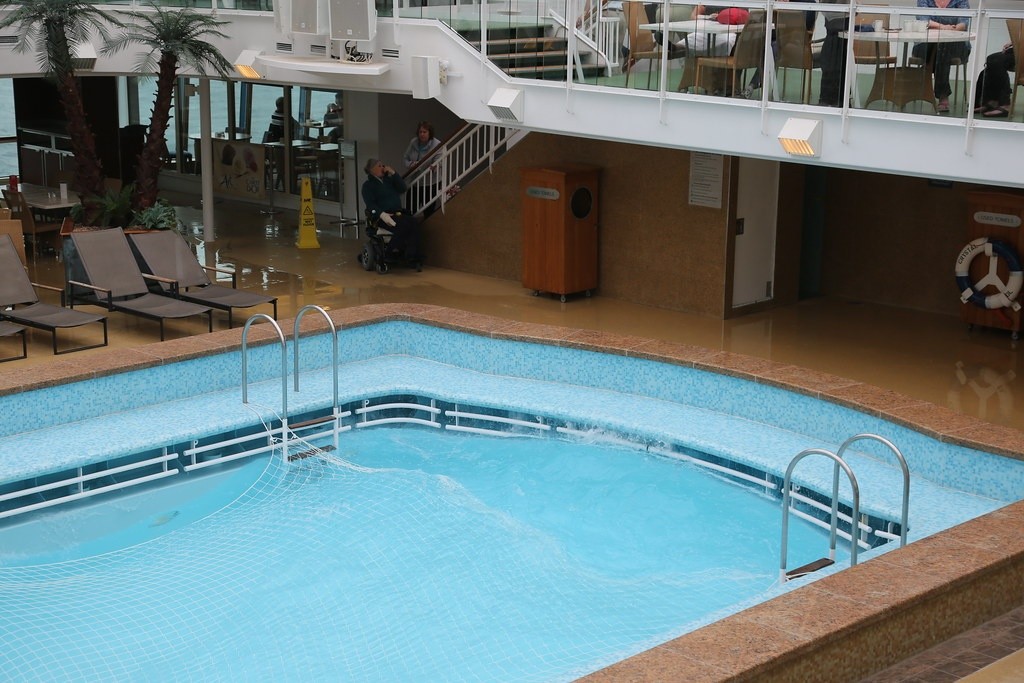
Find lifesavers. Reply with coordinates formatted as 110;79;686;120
954;236;1024;311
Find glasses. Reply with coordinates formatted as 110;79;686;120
373;164;386;168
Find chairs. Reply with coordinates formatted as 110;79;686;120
1;189;61;266
623;2;687;89
130;229;278;330
775;4;1024;123
0;233;109;355
695;9;769;96
70;227;213;343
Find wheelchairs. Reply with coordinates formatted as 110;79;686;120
361;210;427;273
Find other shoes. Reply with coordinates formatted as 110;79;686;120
408;257;418;268
388;247;402;259
739;86;753;98
937;100;951;112
654;32;675;50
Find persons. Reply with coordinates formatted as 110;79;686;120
326;92;343;143
620;4;659;71
361;158;424;272
653;5;750;56
968;41;1015;117
912;0;971;112
272;96;300;137
740;0;817;99
402;121;446;218
818;0;857;108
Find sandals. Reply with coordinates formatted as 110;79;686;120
984;106;1011;117
971;104;997;114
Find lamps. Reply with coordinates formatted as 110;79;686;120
487;88;524;123
233;49;268;80
777;117;823;158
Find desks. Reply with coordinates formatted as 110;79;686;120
636;21;777;89
185;132;251;175
1;181;84;253
262;140;340;197
836;28;978;111
298;119;338;142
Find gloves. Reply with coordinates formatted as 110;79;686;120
378;212;396;227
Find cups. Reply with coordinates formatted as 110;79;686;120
9;175;18;193
